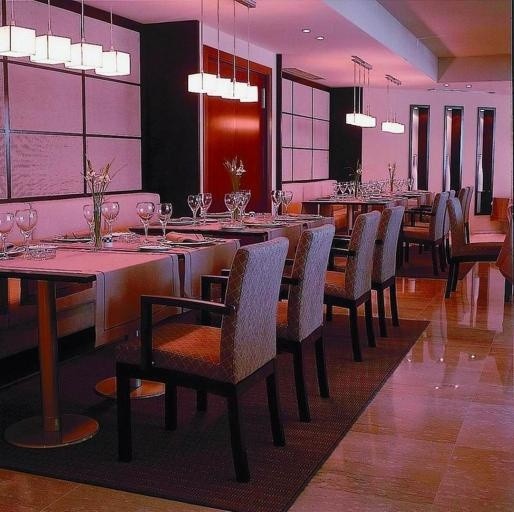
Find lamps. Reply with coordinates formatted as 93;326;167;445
0;1;131;77
381;72;405;135
186;0;259;104
344;55;378;131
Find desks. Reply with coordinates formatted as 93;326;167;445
130;210;335;325
0;232;242;458
495;196;514;304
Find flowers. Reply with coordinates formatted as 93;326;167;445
384;161;398;192
223;156;247;191
350;160;365;199
78;151;130;247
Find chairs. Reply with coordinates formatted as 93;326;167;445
280;177;475;277
446;196;505;298
115;232;290;484
199;223;335;424
322;204;405;341
277;209;380;362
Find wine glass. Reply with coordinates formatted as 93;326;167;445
186;190;293;225
330;177;413;201
100;201;118;239
14;209;38;259
0;214;12;260
83;204;96;245
155;202;172;243
135;201;154;245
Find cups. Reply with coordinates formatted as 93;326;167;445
119;232;142;249
103;235;113;247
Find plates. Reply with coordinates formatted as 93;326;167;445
139;246;170;251
159;221;197;225
285;214;321;219
220;227;242;230
56;237;90;242
404;193;421;197
167;241;213;245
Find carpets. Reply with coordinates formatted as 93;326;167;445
1;295;433;512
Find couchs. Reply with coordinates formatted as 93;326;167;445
0;188;165;367
